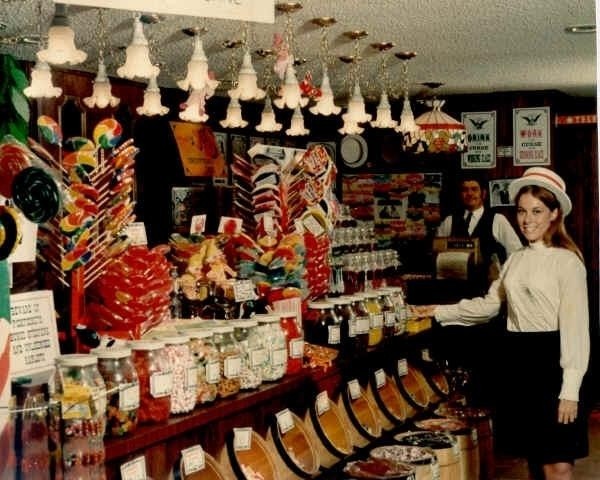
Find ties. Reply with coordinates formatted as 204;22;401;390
465;210;473;231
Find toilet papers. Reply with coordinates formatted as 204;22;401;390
437;250;469;279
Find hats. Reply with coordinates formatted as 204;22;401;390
508;164;572;215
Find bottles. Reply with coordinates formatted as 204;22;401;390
50;306;307;443
328;225;403;297
305;285;407;368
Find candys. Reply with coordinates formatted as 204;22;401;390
0;114;337;474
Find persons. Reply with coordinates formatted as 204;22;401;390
492;183;502;205
407;166;592;480
431;173;523;273
500;183;510;205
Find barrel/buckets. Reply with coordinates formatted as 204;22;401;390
370;445;439;480
433;403;496;476
361;373;413;437
165;448;228;480
413;416;479;479
393;365;430;418
338;459;419;480
213;431;282;480
260;412;320;480
328;386;383;451
299;399;354;477
418;359;451;401
395;428;463;480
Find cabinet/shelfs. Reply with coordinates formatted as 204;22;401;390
69;263;474;480
